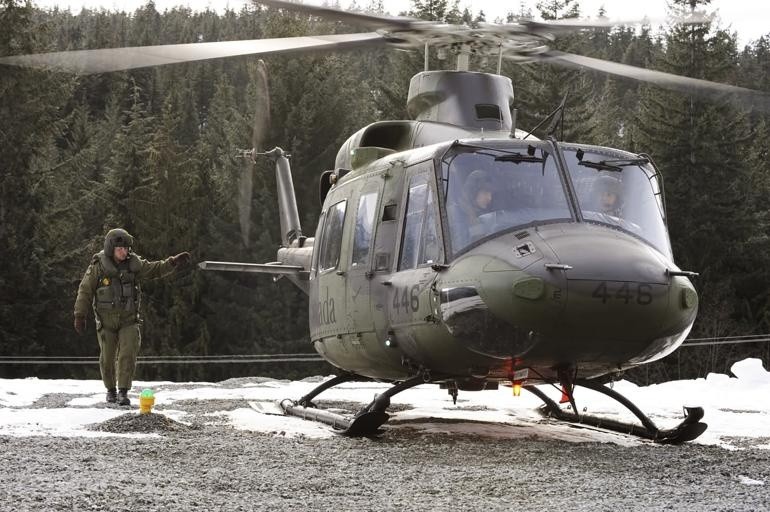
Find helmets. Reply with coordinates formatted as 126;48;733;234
104;229;133;257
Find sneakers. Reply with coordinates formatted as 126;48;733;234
119;390;130;404
106;390;117;402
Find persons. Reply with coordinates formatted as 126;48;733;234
73;227;192;405
590;177;625;217
426;170;499;262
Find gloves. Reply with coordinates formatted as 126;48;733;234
75;317;85;334
169;252;191;267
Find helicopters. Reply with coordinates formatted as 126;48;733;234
1;0;765;444
